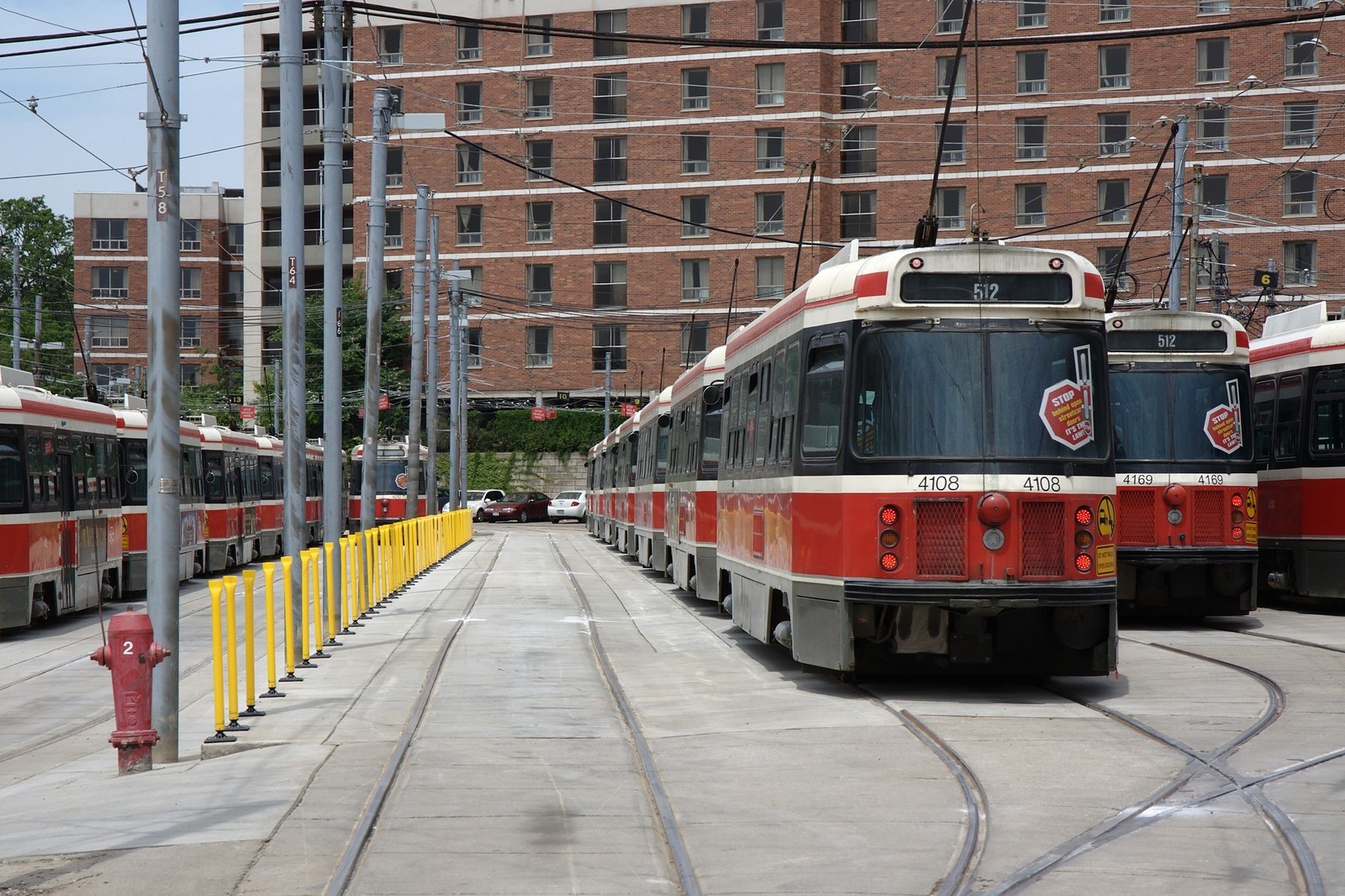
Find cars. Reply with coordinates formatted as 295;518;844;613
437;488;451;514
442;489;506;522
547;490;587;524
483;492;552;524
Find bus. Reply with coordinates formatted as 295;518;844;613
0;365;437;631
586;238;1345;678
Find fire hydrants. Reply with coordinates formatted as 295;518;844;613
89;605;172;777
